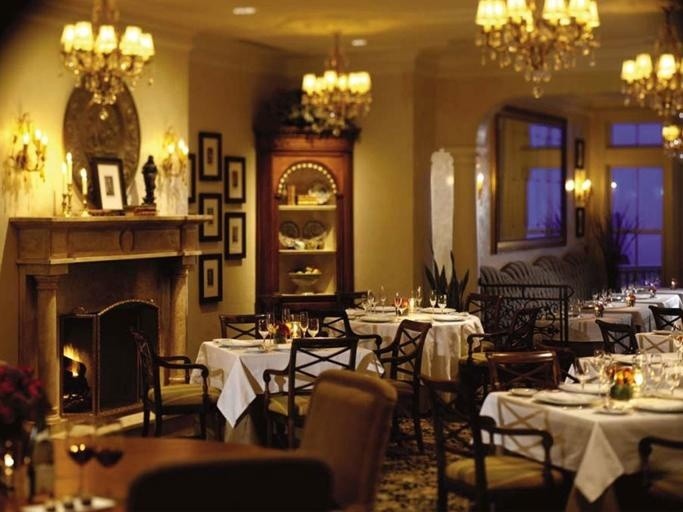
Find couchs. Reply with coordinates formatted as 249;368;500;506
479;248;608;312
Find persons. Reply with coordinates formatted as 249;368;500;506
141;154;159;204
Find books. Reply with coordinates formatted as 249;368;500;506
286;184;291;204
297;201;319;205
291;185;296;204
297;194;317;200
124;211;157;217
87;208;125;217
123;204;157;211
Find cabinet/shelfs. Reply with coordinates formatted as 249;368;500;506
253;98;357;314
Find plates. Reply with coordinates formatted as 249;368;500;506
218;338;258;348
307;179;333;204
508;382;609;407
278;220;328;249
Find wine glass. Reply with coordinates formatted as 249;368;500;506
247;307;337;352
346;283;468;322
25;416;130;512
573;319;683;414
558;277;661;318
289;272;323;295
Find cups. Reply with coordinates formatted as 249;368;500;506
286;182;297;205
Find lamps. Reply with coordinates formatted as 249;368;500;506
6;112;49;182
475;0;683;157
161;126;189;182
302;30;370;137
58;1;156;120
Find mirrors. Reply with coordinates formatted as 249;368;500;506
492;105;566;254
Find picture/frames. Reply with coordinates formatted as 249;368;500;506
188;131;245;305
575;208;585;237
92;156;127;213
575;140;584;168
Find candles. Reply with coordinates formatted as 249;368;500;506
66;152;88;195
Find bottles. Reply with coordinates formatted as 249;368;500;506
668;278;678;290
